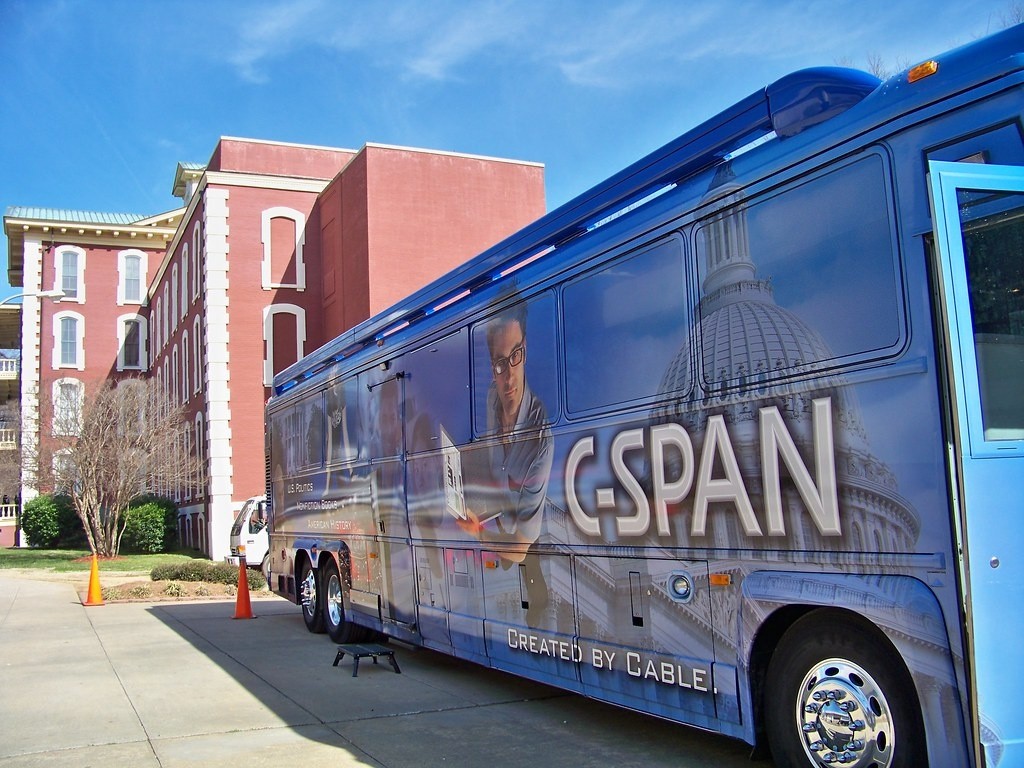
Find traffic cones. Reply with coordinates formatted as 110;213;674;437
231;560;258;619
82;555;106;606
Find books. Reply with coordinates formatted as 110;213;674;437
441;432;467;521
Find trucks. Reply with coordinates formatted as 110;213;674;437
224;494;271;579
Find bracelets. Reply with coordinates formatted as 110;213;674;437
350;471;357;478
479;525;483;544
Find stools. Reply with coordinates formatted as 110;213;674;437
332;644;402;678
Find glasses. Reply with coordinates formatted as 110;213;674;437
492;334;523;375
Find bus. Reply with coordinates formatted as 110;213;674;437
264;23;1024;768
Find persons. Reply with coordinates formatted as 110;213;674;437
413;412;477;616
3;495;10;517
322;364;359;497
456;280;554;631
366;374;446;615
14;495;19;515
274;464;285;515
